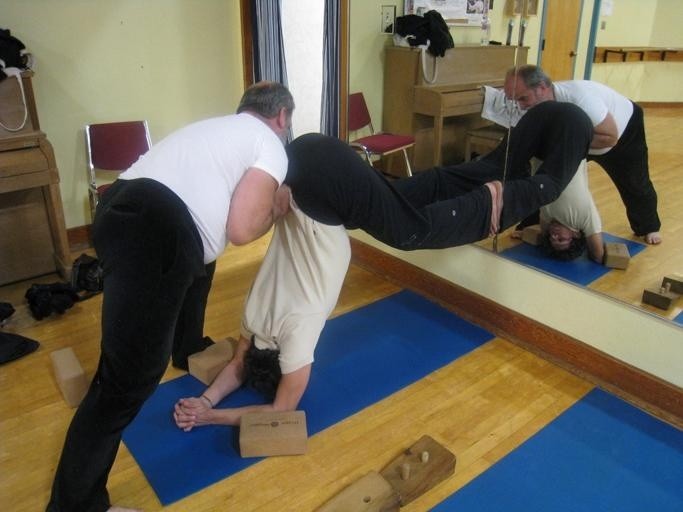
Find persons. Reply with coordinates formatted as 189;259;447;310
170;133;499;434
43;78;296;512
475;99;606;265
502;64;662;246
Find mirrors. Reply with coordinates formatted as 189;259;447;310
343;1;681;331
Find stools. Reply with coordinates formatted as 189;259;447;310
465;124;508;165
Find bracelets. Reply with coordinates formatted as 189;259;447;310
201;395;213;409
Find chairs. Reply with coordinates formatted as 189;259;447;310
82;119;151;221
346;90;417;180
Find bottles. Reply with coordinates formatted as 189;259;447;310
479;16;490;46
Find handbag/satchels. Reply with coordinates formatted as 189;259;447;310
393;33;439;85
0;50;37;132
